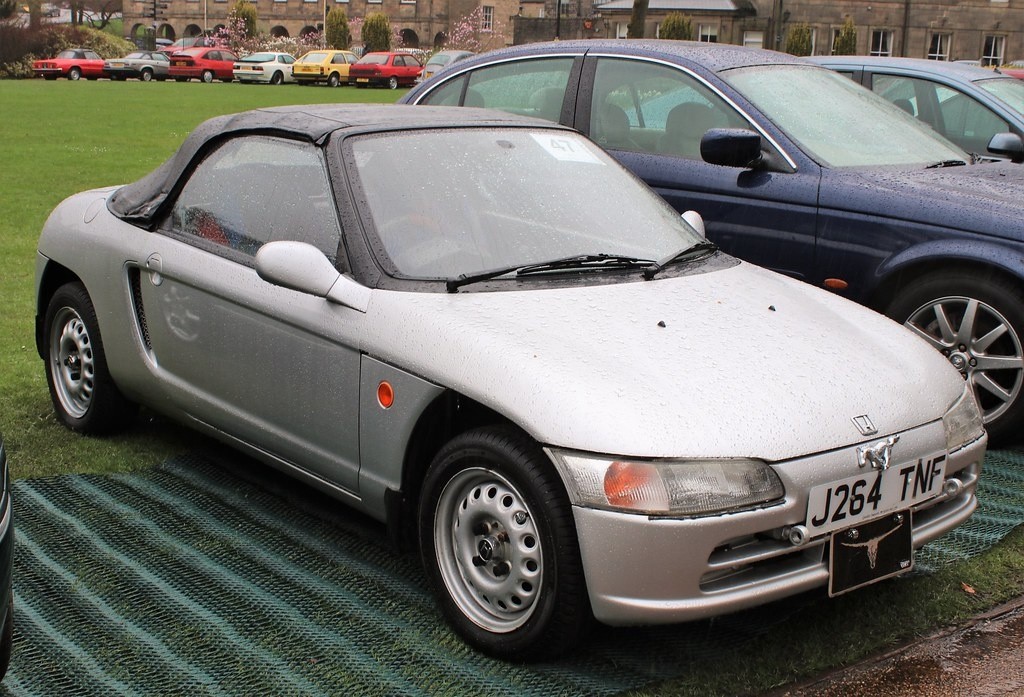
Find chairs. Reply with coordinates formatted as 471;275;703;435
892;98;932;128
655;99;724;157
446;87;485;108
597;104;644;150
528;87;564;121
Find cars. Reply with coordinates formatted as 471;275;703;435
34;101;989;657
291;49;361;87
623;54;1023;170
32;49;111;81
41;3;61;18
393;38;1024;442
233;52;298;85
414;50;488;87
102;51;176;82
168;46;240;83
157;38;233;59
155;38;174;49
348;52;426;90
